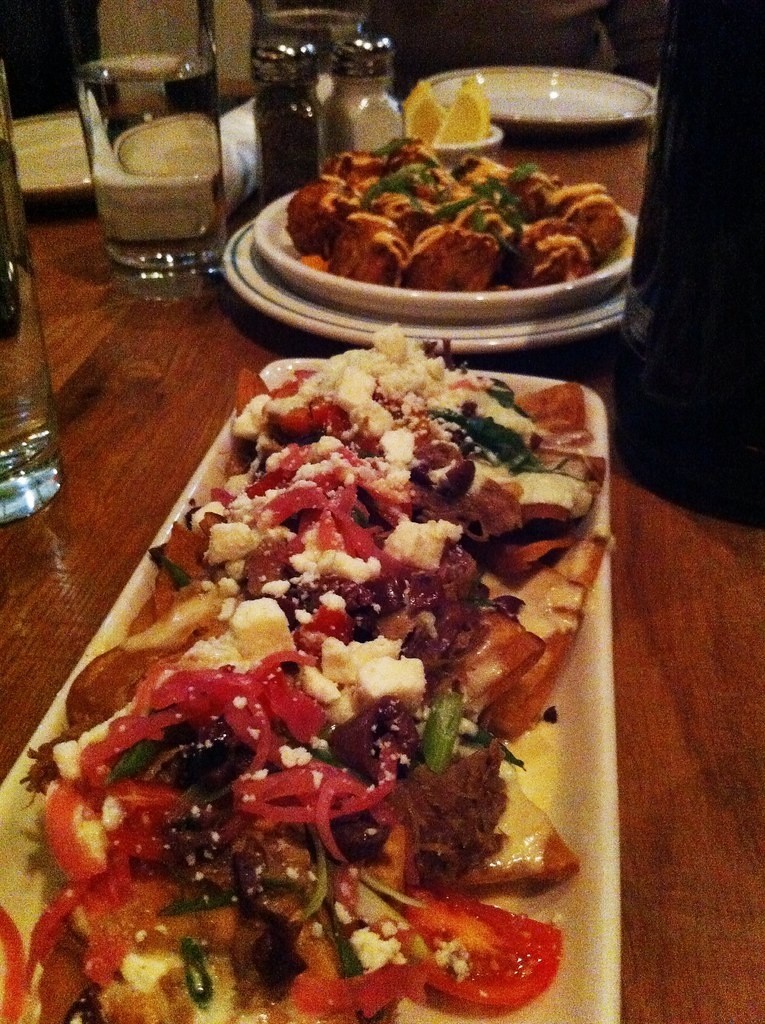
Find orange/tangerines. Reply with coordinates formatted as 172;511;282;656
402;76;489;146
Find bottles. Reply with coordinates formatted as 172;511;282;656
249;37;323;211
612;0;764;527
321;36;404;158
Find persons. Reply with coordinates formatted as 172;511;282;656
246;0;675;101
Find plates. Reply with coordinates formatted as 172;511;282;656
11;111;95;196
1;358;623;1024
218;190;638;353
420;66;658;133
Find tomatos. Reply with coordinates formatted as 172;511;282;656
45;782;111;882
394;884;564;1010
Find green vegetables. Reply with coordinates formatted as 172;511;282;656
107;684;526;1022
363;135;540;246
431;364;545;474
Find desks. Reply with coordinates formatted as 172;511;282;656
0;116;765;1024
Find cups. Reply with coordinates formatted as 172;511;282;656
0;56;66;526
430;125;504;169
60;0;227;270
262;10;367;77
